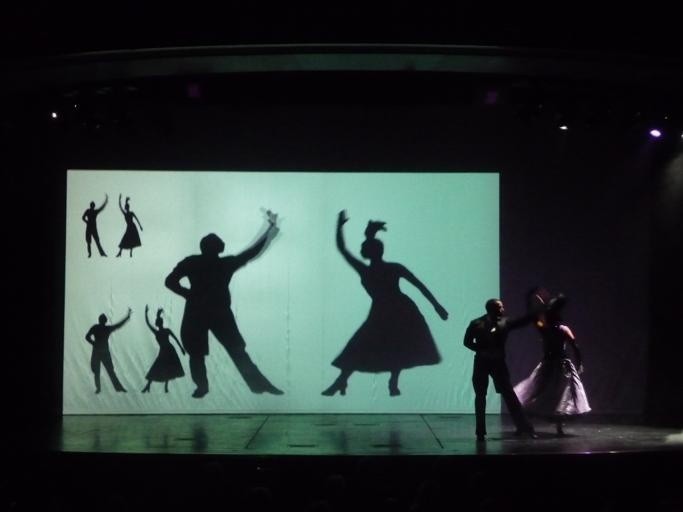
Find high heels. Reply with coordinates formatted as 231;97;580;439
387;378;401;397
320;379;348;397
140;383;168;394
115;254;132;258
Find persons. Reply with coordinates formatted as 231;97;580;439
82;193;109;259
464;291;546;438
512;287;593;436
141;304;185;393
116;193;142;259
84;306;134;394
162;208;287;398
319;209;449;397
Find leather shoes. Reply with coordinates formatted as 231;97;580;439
191;385;209;399
87;253;108;258
251;384;284;395
475;427;565;442
95;386;127;395
